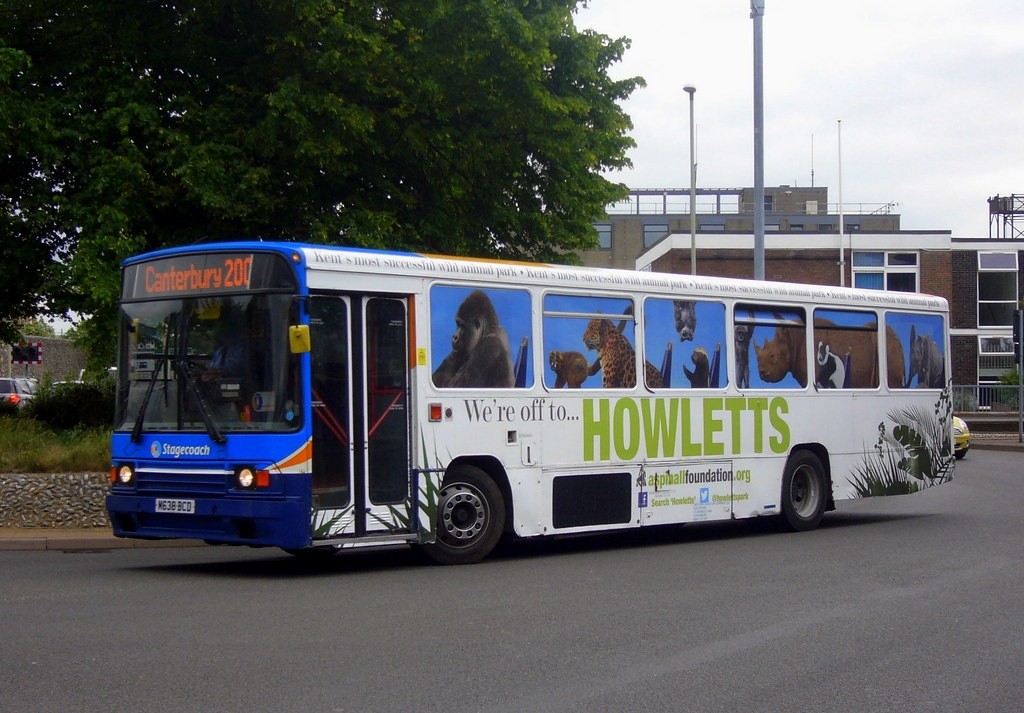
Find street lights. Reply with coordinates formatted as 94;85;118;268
684;86;696;277
837;119;847;288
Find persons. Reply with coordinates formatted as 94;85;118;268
199;324;249;382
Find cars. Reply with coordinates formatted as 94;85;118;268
953;415;971;459
0;366;117;413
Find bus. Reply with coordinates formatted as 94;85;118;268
105;240;955;568
